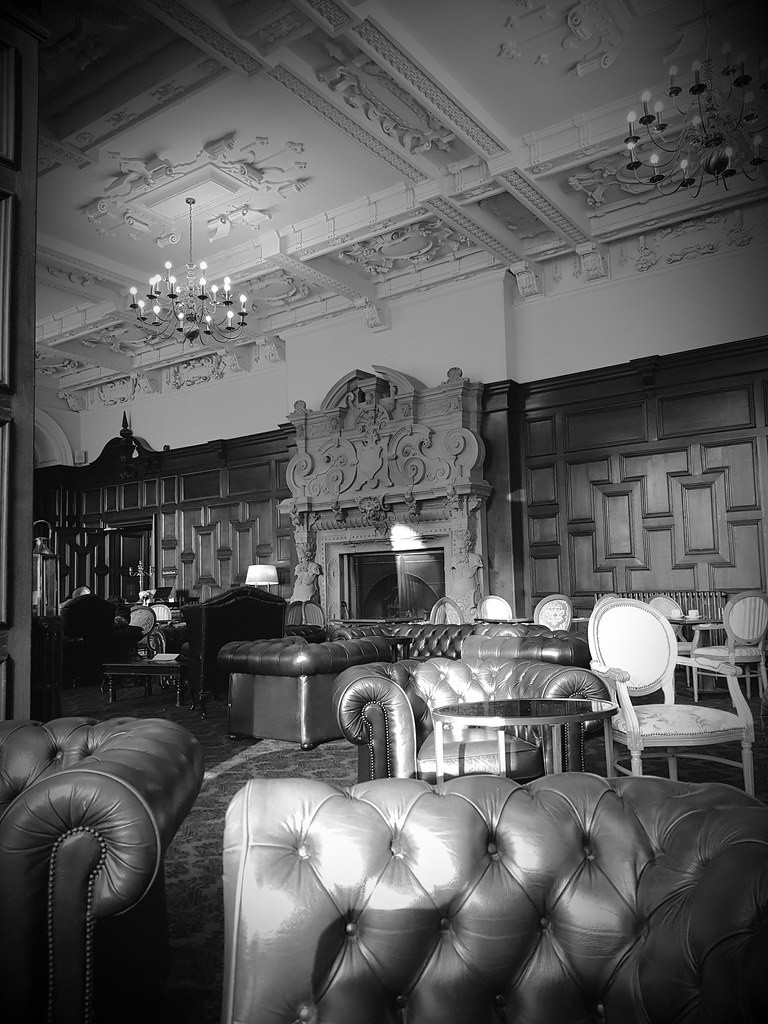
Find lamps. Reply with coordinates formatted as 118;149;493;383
130;194;249;349
129;560;151;598
245;565;280;593
623;3;767;199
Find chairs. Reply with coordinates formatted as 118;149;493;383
534;595;575;636
645;595;692;686
694;590;768;704
286;601;303;626
428;595;465;626
303;599;326;627
588;597;756;798
475;595;513;619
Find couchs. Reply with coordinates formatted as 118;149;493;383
221;771;768;1024
0;714;205;1024
284;624;329;643
332;656;612;778
334;622;592;661
217;637;394;752
29;586;286;711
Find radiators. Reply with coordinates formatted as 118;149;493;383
592;591;729;671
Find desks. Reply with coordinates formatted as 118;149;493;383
329;619;423;628
474;617;591;625
330;634;414;659
433;696;619;778
665;618;725;691
